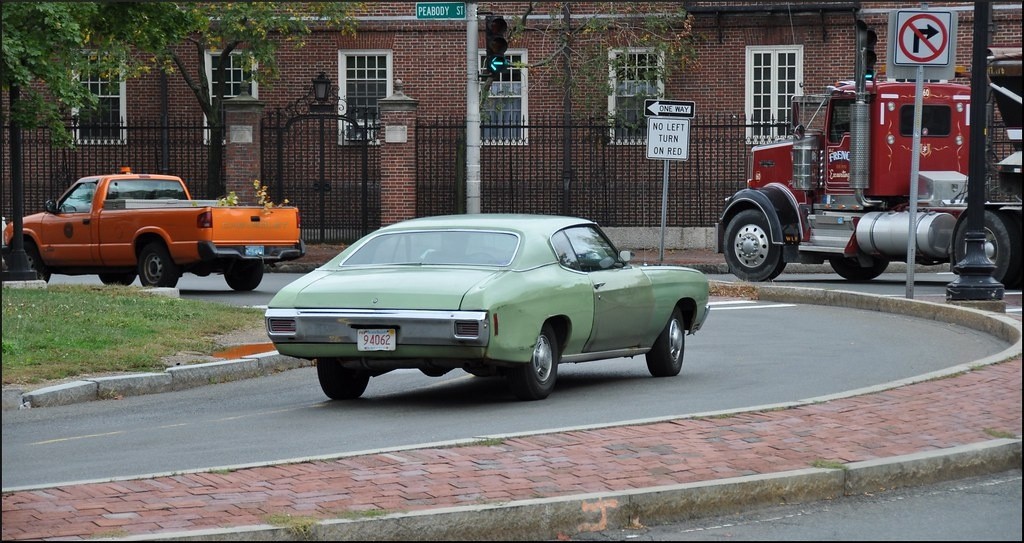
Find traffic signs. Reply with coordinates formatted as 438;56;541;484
416;2;466;20
644;99;695;119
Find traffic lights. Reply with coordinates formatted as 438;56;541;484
486;15;508;73
854;30;878;81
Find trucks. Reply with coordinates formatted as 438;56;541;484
715;19;1024;283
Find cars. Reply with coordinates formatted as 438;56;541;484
264;214;710;400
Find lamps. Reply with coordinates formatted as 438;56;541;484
312;71;331;102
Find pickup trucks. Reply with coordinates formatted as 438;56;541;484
2;173;306;292
